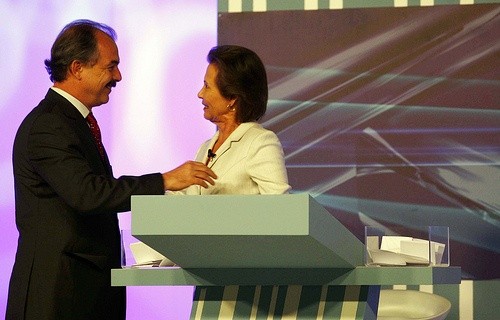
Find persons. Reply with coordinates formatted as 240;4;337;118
8;18;217;320
168;46;292;195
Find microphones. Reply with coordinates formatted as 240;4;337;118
206;148;216;159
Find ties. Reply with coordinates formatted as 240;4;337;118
87;113;105;162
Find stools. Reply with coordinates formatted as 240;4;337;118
377;290;452;320
